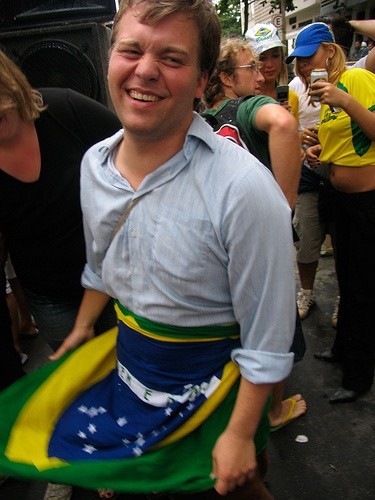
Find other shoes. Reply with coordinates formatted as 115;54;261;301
45;483;72;500
19;323;39;335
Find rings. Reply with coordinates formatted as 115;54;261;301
306;135;309;140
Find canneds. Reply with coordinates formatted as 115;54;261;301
311;68;328;101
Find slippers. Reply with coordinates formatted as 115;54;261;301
270;397;304;433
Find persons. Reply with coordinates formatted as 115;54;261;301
0;48;124;500
0;0;299;500
197;20;375;430
4;251;39;364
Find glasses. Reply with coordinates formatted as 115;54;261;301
226;64;258;73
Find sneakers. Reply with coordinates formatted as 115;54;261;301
296;288;315;317
333;295;341;325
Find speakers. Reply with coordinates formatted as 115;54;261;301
0;25;115;111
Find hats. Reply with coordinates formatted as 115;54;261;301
245;23;287;56
285;22;334;64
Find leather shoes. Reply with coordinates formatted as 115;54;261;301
312;348;345;363
330;386;356;402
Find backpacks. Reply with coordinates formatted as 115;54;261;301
200;93;254;156
